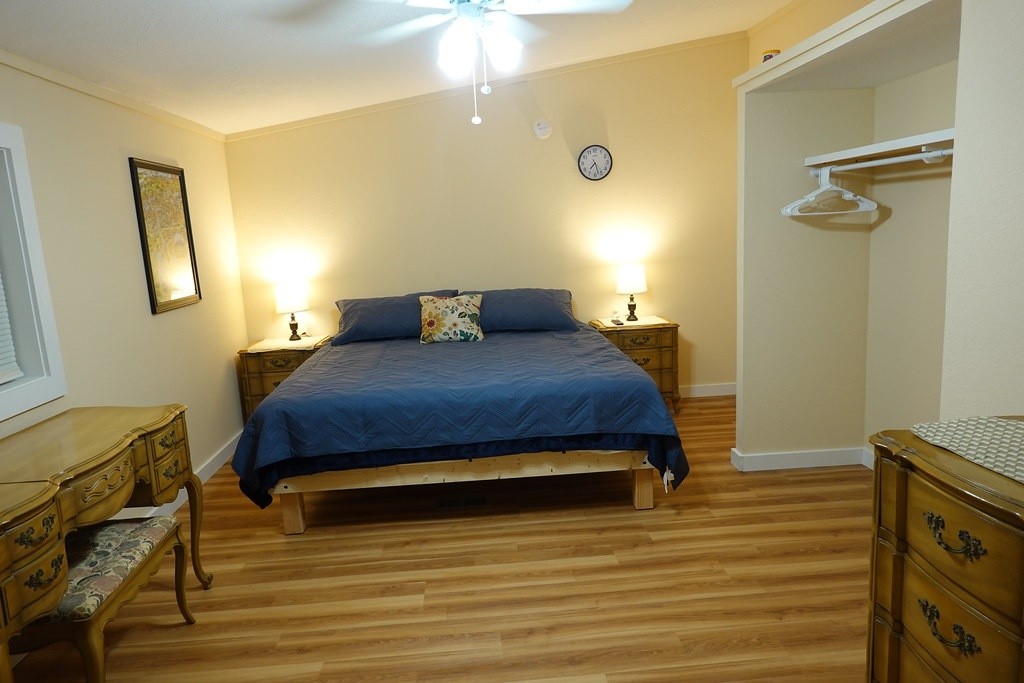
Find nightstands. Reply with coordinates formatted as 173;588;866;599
588;315;681;414
237;334;331;421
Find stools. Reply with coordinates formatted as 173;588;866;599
7;515;198;683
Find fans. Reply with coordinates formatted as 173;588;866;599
372;0;632;41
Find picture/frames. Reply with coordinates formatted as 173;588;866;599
128;157;202;315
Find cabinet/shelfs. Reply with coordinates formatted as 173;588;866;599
730;1;1024;471
866;415;1024;683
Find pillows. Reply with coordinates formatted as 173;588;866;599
419;293;485;345
458;289;581;335
331;290;458;346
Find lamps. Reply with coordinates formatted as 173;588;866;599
276;281;309;340
615;264;647;321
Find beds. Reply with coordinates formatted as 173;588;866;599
230;317;691;535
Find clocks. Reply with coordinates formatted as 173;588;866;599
577;145;613;181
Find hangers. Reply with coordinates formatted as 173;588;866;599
780;164;877;216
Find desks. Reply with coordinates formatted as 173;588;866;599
0;403;214;683
